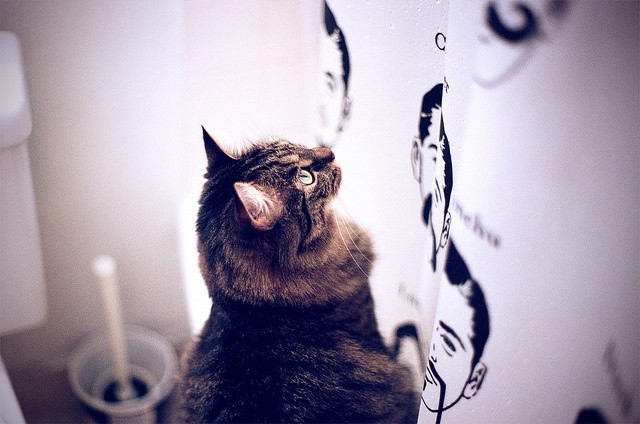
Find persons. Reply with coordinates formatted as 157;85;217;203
429;132;454;274
420;236;492;423
322;0;352;149
409;82;444;226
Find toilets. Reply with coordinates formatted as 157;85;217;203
0;31;47;424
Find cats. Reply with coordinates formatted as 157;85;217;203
151;124;426;423
